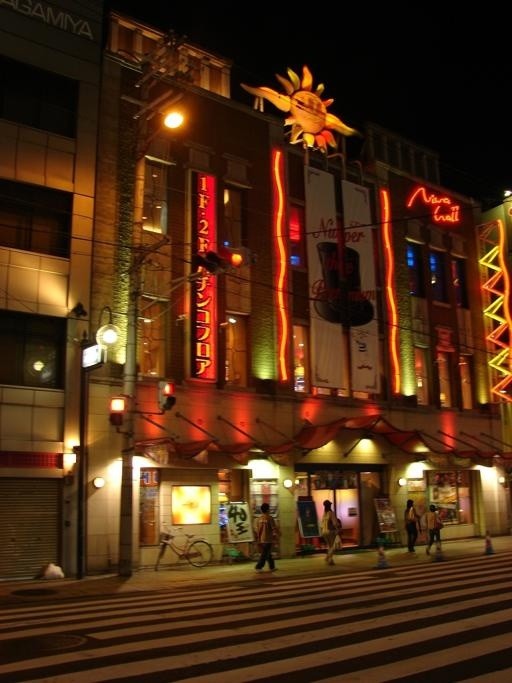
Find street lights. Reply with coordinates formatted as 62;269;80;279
76;304;124;579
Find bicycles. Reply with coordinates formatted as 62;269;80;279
155;521;214;571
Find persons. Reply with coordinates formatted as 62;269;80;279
318;498;344;567
402;497;422;553
253;502;281;573
419;503;444;556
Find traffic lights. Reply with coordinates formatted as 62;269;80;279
157;380;176;411
193;246;252;274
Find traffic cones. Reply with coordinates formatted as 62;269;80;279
373;545;390;568
431;541;446;562
483;529;496;555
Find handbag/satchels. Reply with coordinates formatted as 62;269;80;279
409;507;419;521
434;512;443;530
327;511;342;531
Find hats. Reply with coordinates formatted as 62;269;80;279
323;499;333;505
